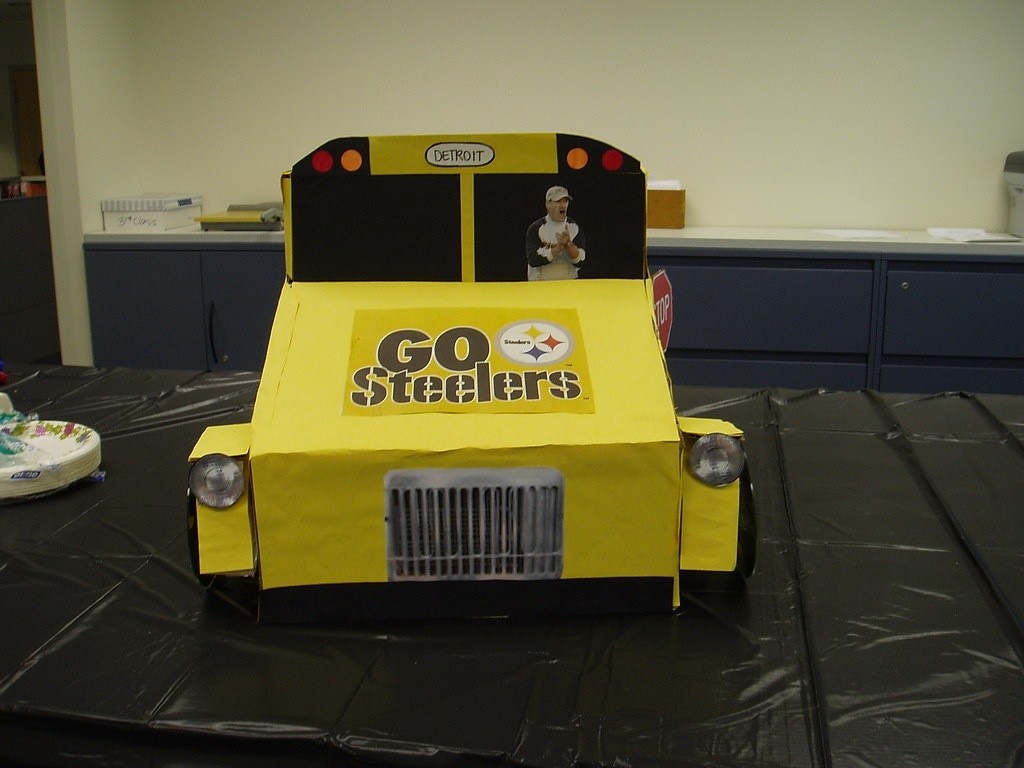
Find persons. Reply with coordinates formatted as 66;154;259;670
526;186;585;280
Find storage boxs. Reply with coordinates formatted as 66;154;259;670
647;188;685;229
0;175;47;199
101;196;203;232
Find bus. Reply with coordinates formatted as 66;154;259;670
186;130;758;610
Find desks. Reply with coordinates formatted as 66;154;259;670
0;359;1024;768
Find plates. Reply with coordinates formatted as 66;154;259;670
0;419;101;500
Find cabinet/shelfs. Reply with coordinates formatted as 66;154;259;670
0;194;61;363
644;226;1024;395
84;228;286;373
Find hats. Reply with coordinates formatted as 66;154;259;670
545;186;572;202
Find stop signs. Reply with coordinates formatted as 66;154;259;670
650;268;675;354
